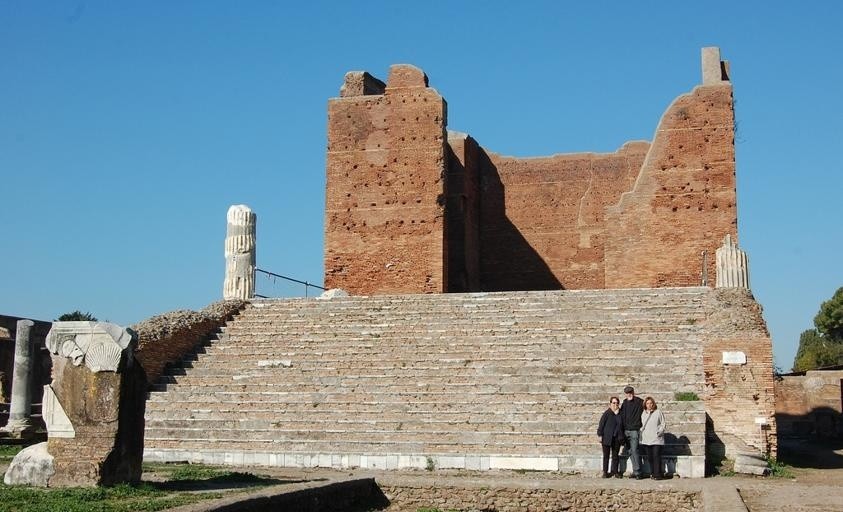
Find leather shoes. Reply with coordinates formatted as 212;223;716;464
628;473;661;480
615;473;621;479
602;473;608;478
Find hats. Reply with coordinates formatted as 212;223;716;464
623;387;633;393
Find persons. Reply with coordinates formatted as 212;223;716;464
618;386;644;479
596;396;623;478
638;397;665;480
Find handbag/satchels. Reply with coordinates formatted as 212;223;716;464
637;431;641;441
